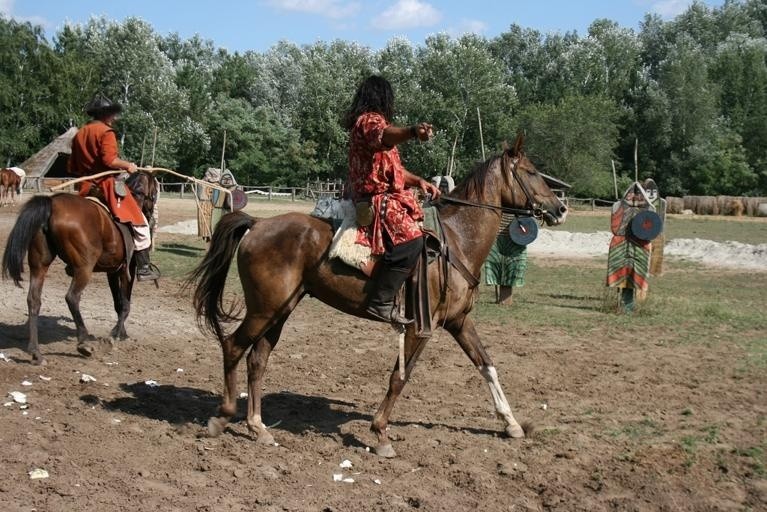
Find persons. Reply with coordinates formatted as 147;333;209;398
347;73;443;326
69;91;160;283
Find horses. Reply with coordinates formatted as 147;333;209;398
5;166;26;203
177;131;569;458
1;169;175;366
0;168;22;207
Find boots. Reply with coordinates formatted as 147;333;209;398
135;248;159;281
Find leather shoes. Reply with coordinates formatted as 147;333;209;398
366;301;414;324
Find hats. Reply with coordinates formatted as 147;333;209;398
87;88;120;116
